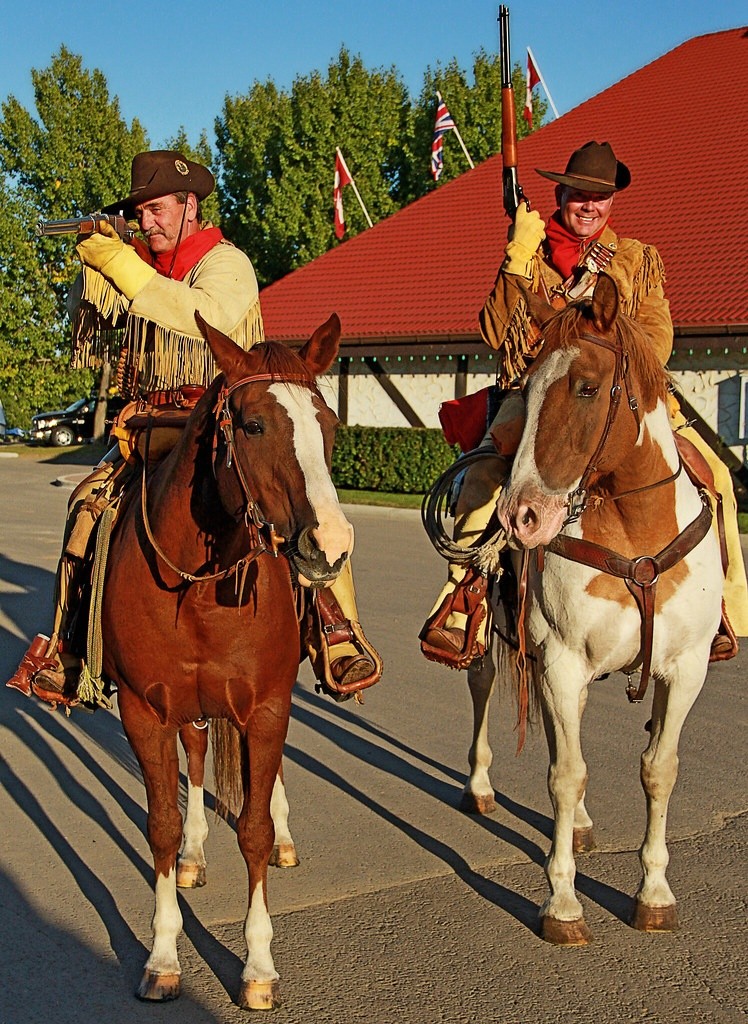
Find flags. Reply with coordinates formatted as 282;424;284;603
430;102;455;179
331;153;353;242
518;53;539;128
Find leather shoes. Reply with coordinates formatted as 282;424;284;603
428;627;486;676
33;666;95;716
711;633;733;656
319;655;372;703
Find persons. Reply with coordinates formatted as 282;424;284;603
424;142;738;659
34;151;375;701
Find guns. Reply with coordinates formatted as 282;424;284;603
34;210;153;267
496;3;531;218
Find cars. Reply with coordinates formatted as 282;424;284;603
29;395;128;446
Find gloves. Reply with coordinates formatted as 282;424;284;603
501;202;546;279
75;220;158;300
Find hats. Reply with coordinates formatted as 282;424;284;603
535;139;632;193
100;150;216;215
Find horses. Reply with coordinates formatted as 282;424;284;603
94;307;355;1014
459;271;725;948
